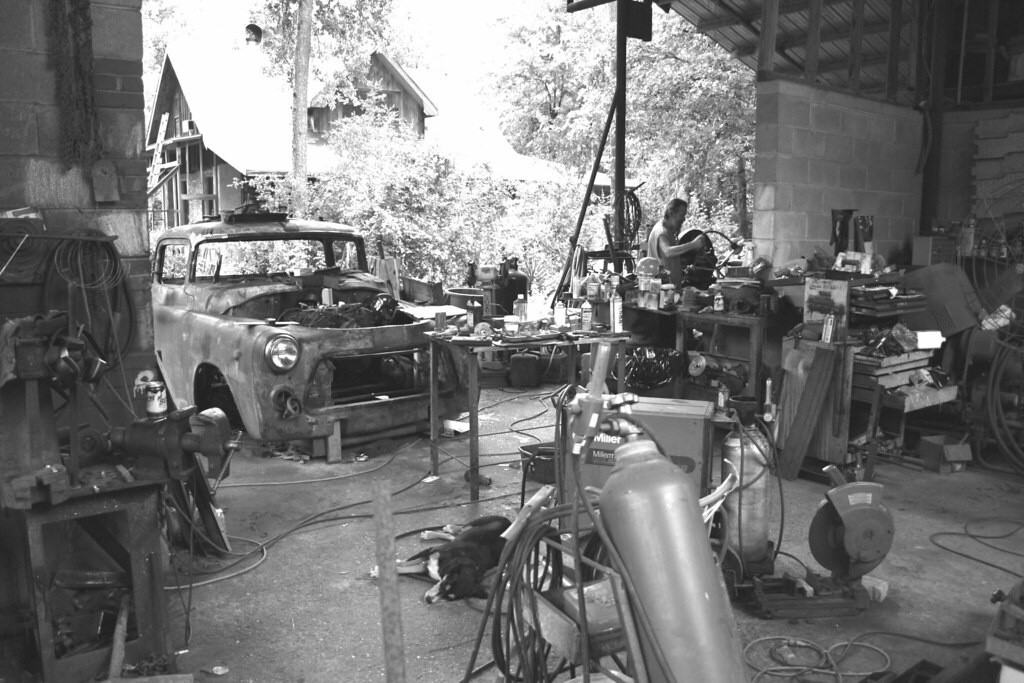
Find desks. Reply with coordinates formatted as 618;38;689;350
423;331;630;501
673;310;790;413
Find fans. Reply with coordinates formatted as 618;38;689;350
958;172;1024;352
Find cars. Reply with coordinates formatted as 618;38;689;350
150;207;478;455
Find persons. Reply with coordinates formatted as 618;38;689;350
647;198;706;286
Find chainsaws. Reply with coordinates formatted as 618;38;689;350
751;464;896;620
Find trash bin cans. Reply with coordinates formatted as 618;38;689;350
444;287;484;309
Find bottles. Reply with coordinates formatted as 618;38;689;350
554;301;566;327
512;294;528;323
571;265;671;333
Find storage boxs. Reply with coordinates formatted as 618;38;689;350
564;394;715;528
918;433;974;473
911;235;956;267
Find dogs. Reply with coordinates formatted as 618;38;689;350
370;516;511;605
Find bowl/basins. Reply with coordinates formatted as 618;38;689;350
446;288;484;309
